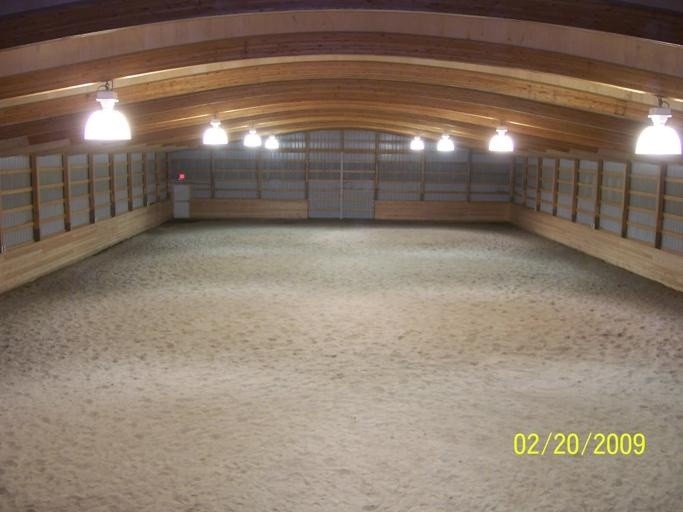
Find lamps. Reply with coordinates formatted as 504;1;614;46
82;76;133;142
200;118;228;147
242;127;282;151
487;123;513;153
410;132;455;154
633;95;683;157
